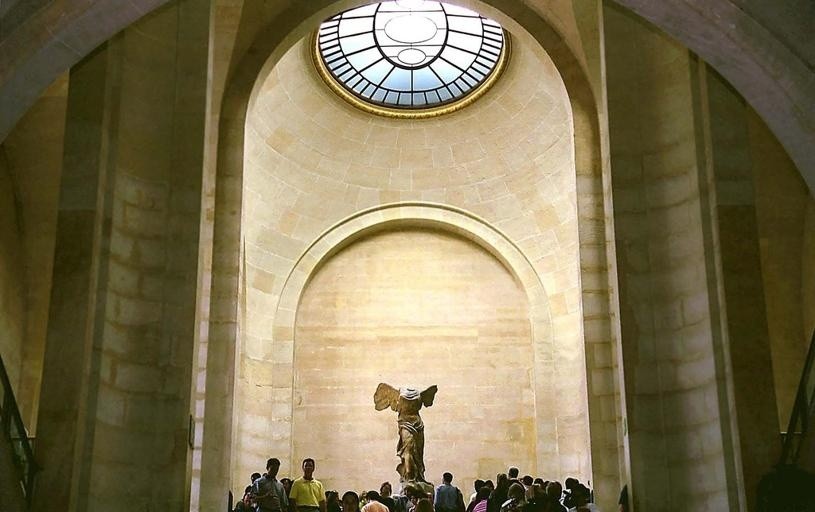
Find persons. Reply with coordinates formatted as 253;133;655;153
396;387;426;484
325;481;434;511
240;457;327;511
466;467;593;511
435;472;466;511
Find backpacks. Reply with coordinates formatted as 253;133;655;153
563;488;577;509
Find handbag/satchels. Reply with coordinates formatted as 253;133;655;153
456;487;465;512
